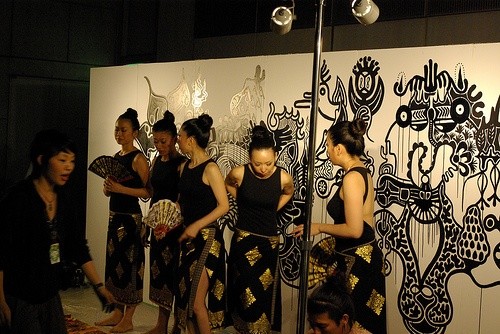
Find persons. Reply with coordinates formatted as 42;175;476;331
94;107;150;334
289;117;386;334
177;115;230;334
140;110;190;334
225;124;294;334
306;271;375;334
0;127;117;334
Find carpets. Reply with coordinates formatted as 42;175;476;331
64;314;107;334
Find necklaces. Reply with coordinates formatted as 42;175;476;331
37;185;55;211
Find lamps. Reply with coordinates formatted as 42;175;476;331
350;0;380;26
270;3;297;37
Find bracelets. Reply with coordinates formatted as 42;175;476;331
94;283;103;289
